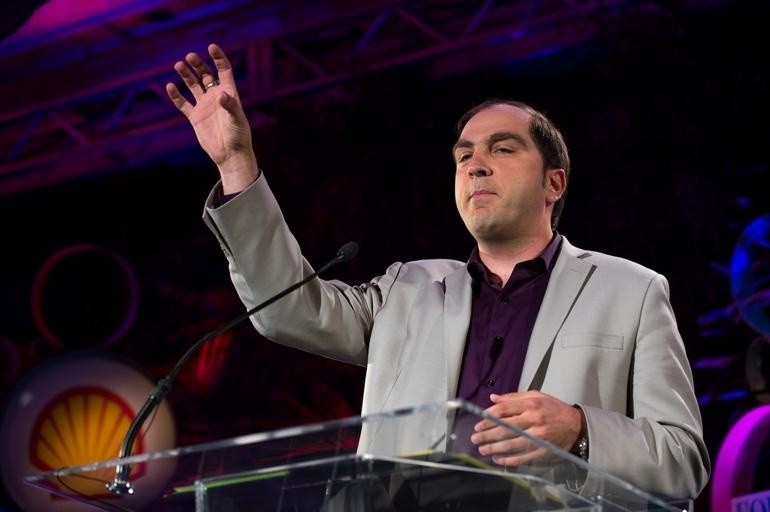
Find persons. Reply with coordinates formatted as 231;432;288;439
164;43;712;511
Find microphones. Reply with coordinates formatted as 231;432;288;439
107;241;359;496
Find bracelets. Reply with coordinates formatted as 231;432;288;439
569;405;591;468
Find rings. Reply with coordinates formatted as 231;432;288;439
204;80;218;90
